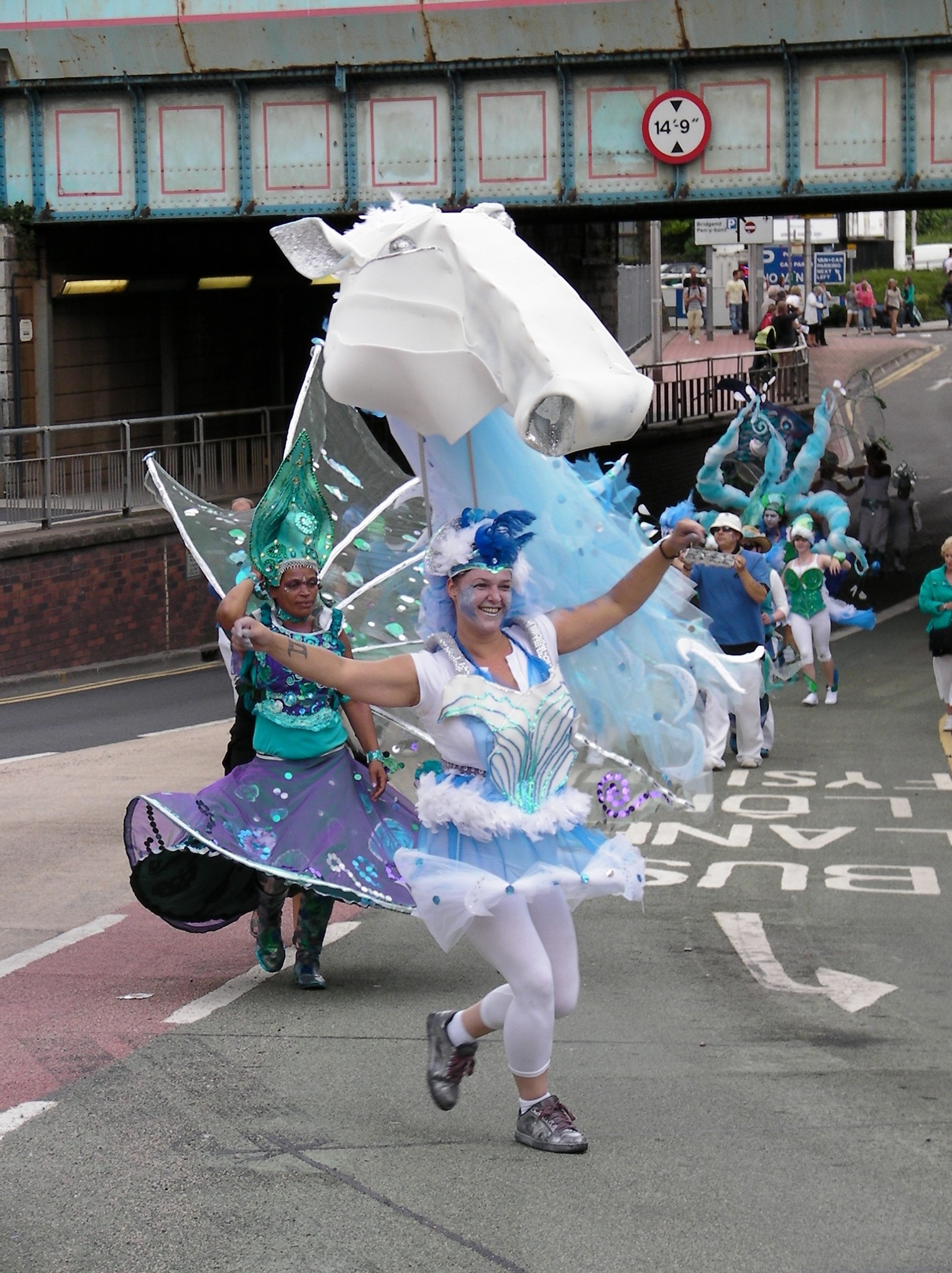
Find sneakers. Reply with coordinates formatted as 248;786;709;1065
425;1010;478;1110
514;1095;588;1153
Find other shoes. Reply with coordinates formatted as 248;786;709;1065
894;556;900;567
292;931;300;949
740;758;758;768
729;732;737;753
897;565;905;571
250;909;259;938
943;715;952;731
760;748;769;758
802;692;818;706
824;685;837;704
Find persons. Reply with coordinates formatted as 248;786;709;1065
672;443;918;771
725;268;832;389
919;536;952;732
941;248;952;329
206;496;257;704
230;506;705;1156
123;430;423;992
897;276;915;328
842;276;878;337
682;268;705;345
883;278;905;336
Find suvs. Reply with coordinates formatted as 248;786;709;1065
660;262;710;274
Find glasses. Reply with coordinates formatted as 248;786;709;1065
713;526;731;532
740;543;757;551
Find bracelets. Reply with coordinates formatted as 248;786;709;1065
245;571;261;592
832;550;846;564
363;750;385;763
846;466;853;480
943;602;948;610
682;563;692;571
768;613;776;625
659;540;680;559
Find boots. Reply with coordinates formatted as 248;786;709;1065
292;892;335;989
250;884;289;972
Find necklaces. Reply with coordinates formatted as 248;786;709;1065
797;552;813;580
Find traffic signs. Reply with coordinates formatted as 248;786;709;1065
814;252;846;284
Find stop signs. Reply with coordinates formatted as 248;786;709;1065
744;221;756;233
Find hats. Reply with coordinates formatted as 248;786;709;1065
790;513;815;545
764;493;785;515
425;508;536;595
742;525;772;553
709;513;743;541
249;428;334;586
